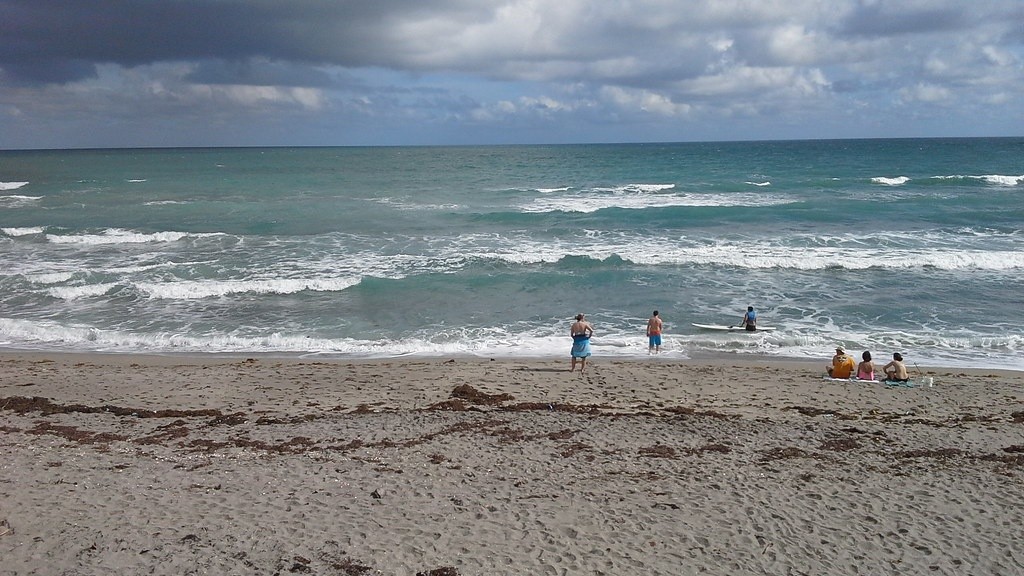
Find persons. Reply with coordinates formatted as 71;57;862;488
569;313;593;371
826;346;855;379
883;353;909;382
647;310;662;351
856;351;875;380
742;306;757;331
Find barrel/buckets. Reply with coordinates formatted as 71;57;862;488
922;376;933;387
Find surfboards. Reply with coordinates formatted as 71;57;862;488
692;323;777;331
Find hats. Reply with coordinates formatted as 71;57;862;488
835;346;846;352
